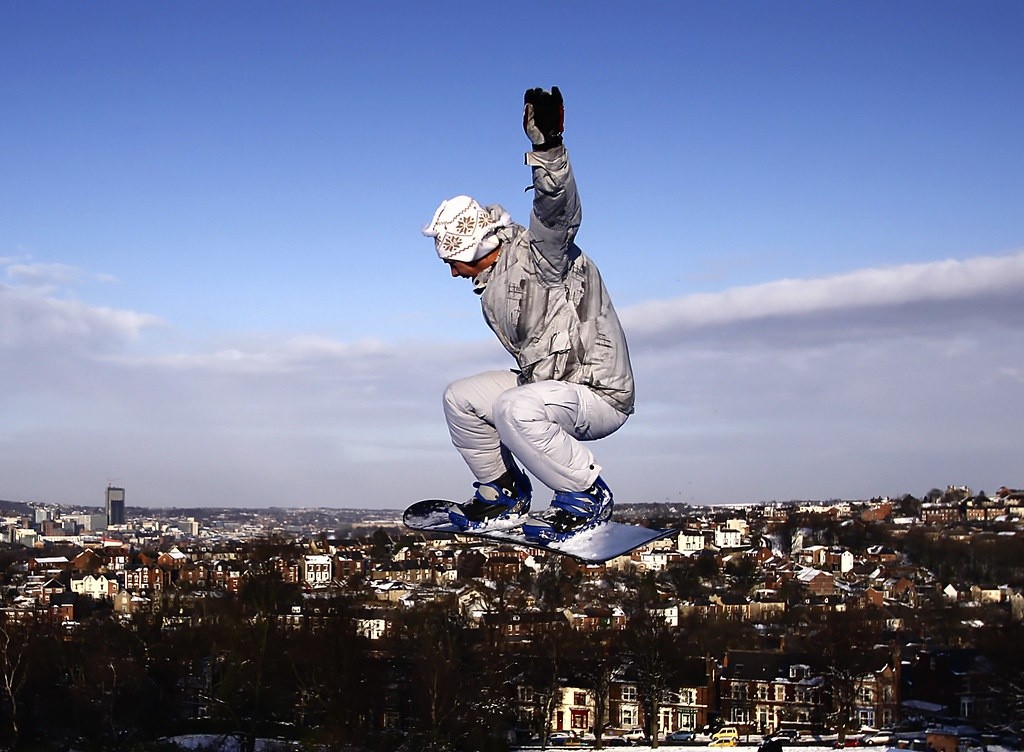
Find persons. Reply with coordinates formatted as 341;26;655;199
421;85;636;541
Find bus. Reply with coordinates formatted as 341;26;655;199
778;719;826;735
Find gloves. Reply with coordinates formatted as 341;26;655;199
522;85;564;152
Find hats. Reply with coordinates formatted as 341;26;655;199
422;195;511;262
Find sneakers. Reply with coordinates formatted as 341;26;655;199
448;469;533;529
522;474;614;545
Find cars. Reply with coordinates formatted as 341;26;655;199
767;737;791;745
665;730;691;742
549;732;572;740
576;732;597;742
708;736;737;746
711;728;740;742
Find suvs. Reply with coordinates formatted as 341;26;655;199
622;729;645;741
762;727;803;742
864;732;898;747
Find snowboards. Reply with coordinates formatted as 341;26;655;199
403;496;678;561
